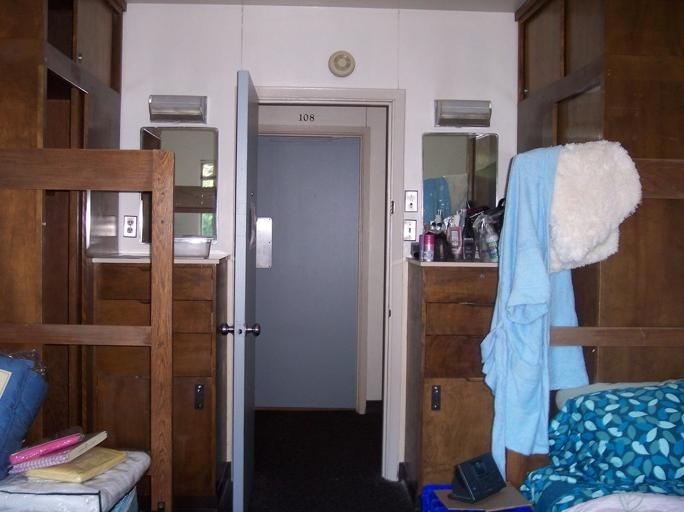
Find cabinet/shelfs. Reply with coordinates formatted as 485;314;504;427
465;134;497;212
514;0;684;383
0;0;127;446
94;252;227;497
405;256;498;497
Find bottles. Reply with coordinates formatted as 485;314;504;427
420;209;476;263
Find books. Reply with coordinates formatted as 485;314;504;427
6;425;127;483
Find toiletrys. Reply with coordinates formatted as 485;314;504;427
419;207;498;263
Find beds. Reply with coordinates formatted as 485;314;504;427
481;158;684;512
0;146;174;512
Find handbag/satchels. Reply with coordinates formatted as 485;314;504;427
447;451;509;504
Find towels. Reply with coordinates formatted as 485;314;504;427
445;173;469;215
482;144;590;481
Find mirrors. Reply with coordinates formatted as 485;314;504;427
422;132;498;224
140;127;218;243
